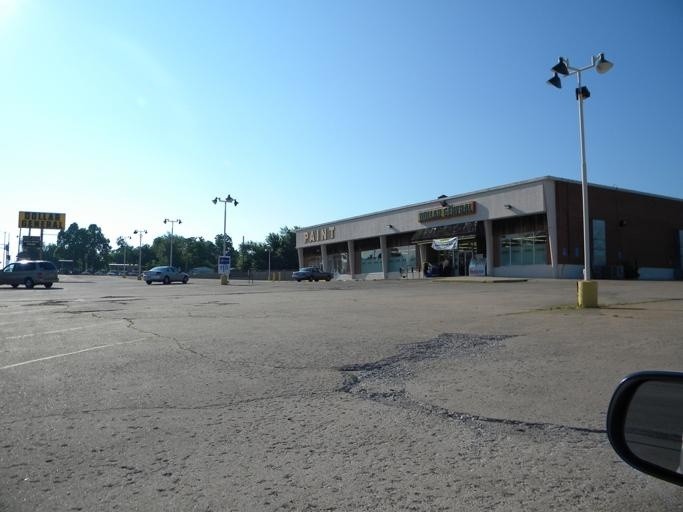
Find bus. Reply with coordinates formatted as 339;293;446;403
108;264;139;276
291;266;333;283
58;260;74;275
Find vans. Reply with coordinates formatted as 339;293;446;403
0;258;60;292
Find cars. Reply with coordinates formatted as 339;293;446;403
163;218;183;266
142;264;192;287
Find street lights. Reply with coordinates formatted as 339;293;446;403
133;229;148;281
120;235;133;279
264;248;275;280
210;194;240;286
544;50;618;310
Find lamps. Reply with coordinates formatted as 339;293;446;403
504;205;511;209
387;224;392;228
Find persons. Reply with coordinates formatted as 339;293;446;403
443;257;450;276
